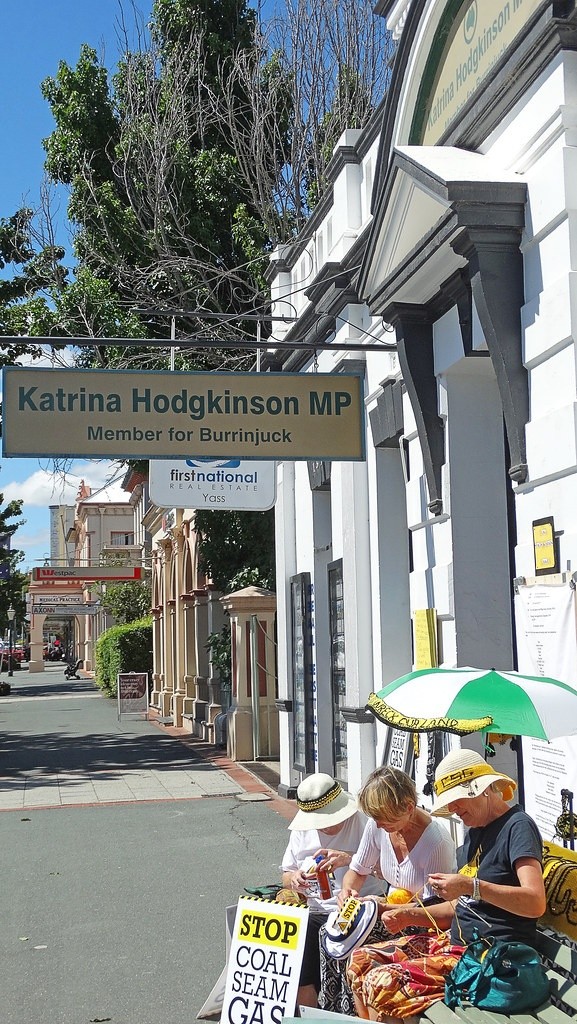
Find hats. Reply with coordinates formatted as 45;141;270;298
428;749;517;819
288;773;361;832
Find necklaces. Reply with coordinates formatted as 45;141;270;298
394;806;417;845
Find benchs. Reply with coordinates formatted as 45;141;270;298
410;839;577;1024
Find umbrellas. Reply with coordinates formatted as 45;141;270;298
365;665;577;762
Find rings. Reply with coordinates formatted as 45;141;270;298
327;861;333;865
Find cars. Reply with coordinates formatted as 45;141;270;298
0;641;29;672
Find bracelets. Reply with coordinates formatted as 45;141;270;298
473;876;481;901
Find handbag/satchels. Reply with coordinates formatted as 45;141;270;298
444;936;549;1014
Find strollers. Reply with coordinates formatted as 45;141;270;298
63;659;83;681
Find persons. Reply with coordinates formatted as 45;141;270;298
318;766;458;1018
279;772;369;1017
346;749;546;1024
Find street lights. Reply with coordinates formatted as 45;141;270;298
6;602;16;676
